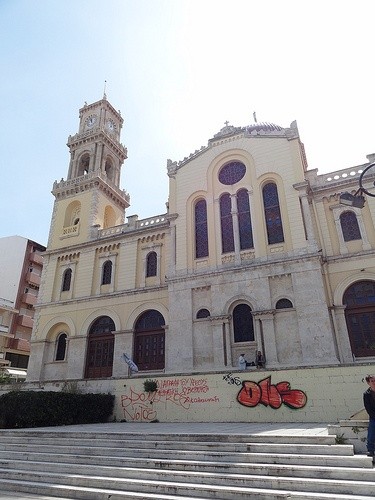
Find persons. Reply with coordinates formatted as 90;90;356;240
363;374;375;456
238;353;247;370
256;351;266;368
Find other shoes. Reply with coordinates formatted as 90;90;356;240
366;452;373;455
